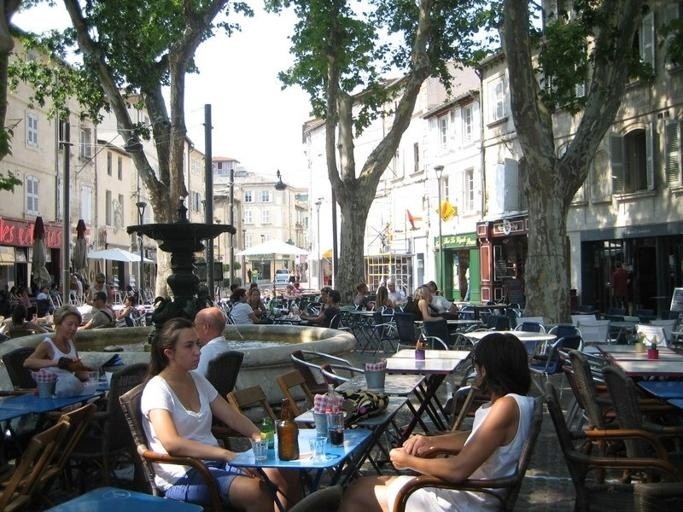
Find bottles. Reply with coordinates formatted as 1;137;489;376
277;397;299;461
327;384;334;398
58;357;92;372
652;335;656;349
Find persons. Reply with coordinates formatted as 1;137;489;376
354;277;470;351
139;315;299;511
225;281;342;329
191;305;231;378
337;332;534;512
0;272;140;340
245;267;302;283
610;261;636;316
21;305;90;381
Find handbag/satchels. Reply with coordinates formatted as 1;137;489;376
324;389;388;428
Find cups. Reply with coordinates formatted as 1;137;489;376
33;314;37;321
327;413;344;448
648;349;658;359
313;413;343;437
272;308;292;320
309;435;327;462
362;307;367;312
88;371;96;394
351;376;358;385
364;371;385;388
251;439;268;461
634;343;646;353
36;383;55;399
414;350;425;360
260;415;274;449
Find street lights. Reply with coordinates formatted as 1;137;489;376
134;202;147;297
433;165;444;290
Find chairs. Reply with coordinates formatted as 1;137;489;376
545;382;682;512
205;352;244;424
602;367;683;481
45;363;151;485
3;346;50;391
0;403;97;501
0;421;70;512
118;375;251;511
569;353;682;448
397;400;544;511
228;384;277;439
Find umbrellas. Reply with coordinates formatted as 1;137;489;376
86;248;154;263
236;238;311;298
72;217;88;272
32;215;51;289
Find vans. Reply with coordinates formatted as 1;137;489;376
274;269;290;282
